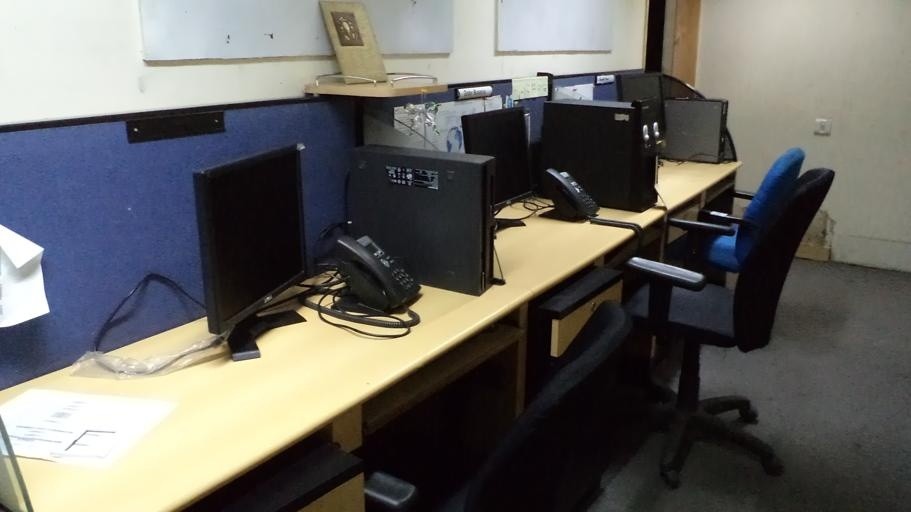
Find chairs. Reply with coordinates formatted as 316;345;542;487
363;301;634;512
697;148;805;274
628;168;834;489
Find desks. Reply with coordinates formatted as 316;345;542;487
0;157;744;512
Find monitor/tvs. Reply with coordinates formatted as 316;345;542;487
192;141;310;361
615;68;667;138
461;105;530;229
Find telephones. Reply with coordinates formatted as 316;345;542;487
543;168;600;221
324;224;421;315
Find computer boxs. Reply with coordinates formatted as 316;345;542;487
660;97;728;164
540;100;659;213
348;144;492;297
